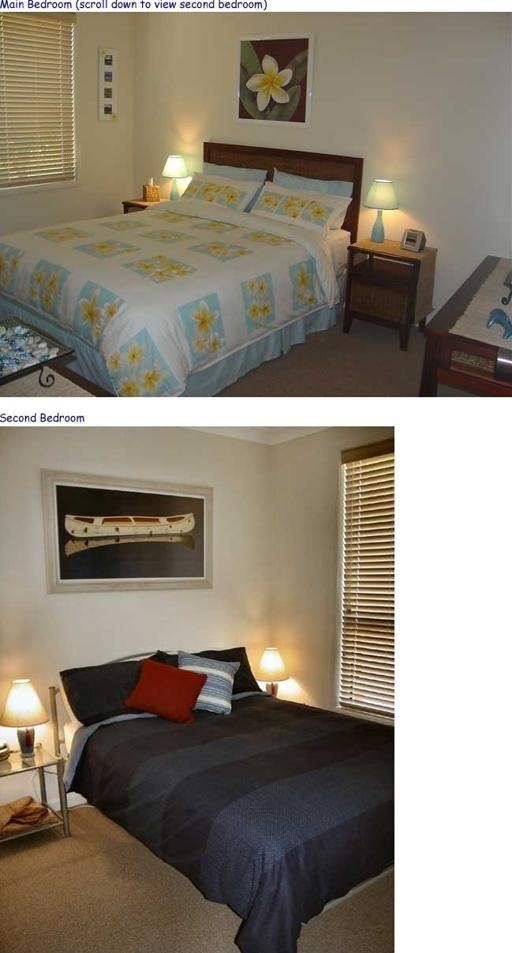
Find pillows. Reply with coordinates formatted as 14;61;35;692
125;657;208;724
56;656;171;727
177;648;242;714
181;170;262;214
273;166;353;230
250;180;352;236
156;647;270;700
201;162;268;212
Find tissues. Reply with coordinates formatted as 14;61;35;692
142;176;161;202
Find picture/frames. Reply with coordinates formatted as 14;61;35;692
231;31;315;129
39;466;214;595
97;46;119;121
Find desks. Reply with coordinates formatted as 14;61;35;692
1;744;70;845
1;309;74;386
419;254;511;396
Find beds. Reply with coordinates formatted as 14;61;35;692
49;641;394;950
0;140;364;396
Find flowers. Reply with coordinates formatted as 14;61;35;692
240;41;309;120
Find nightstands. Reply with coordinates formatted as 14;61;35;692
121;195;168;213
342;238;438;350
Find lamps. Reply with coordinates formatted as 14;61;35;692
365;179;399;241
0;676;50;758
161;154;190;200
255;646;292;697
